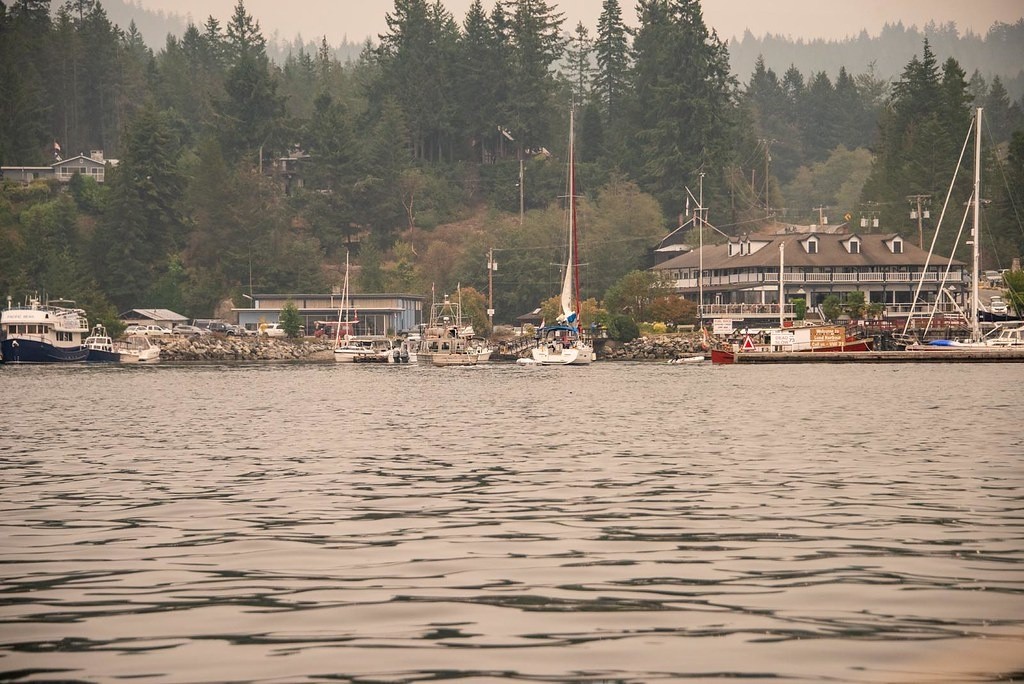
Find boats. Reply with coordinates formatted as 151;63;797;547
334;252;417;364
82;323;121;362
416;281;494;365
711;241;874;365
0;289;91;365
117;335;160;361
113;340;139;362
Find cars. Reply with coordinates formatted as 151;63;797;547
989;295;1002;305
989;302;1009;316
147;325;171;335
173;325;203;336
980;268;1010;287
124;325;148;336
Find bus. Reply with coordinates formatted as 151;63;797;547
313;321;354;338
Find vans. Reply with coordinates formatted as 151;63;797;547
258;323;289;338
209;322;247;336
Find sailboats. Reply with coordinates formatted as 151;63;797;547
880;106;1024;352
531;111;594;366
